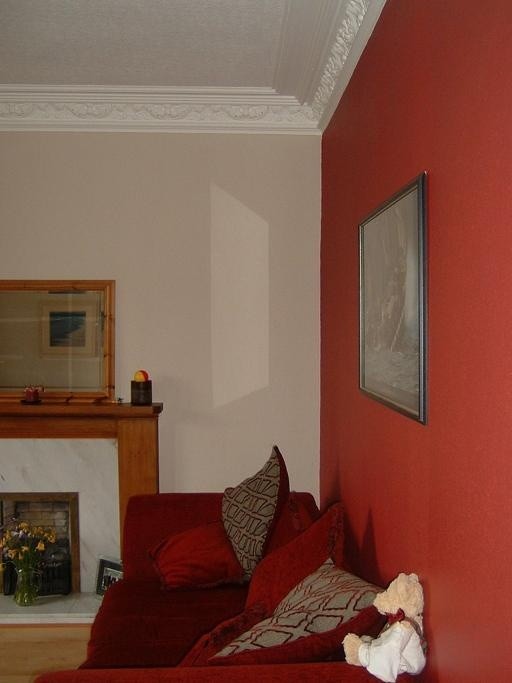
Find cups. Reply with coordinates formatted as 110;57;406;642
131;379;152;406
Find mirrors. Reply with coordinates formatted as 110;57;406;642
0;278;117;406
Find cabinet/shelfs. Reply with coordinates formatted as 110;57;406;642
2;401;165;682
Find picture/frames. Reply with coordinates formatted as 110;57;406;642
357;170;431;425
93;556;126;600
38;297;102;361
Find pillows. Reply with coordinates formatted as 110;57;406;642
243;500;346;616
177;606;273;664
218;445;293;577
145;522;251;588
205;556;395;662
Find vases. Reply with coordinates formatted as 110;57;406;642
8;566;43;608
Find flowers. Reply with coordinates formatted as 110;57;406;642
0;517;66;576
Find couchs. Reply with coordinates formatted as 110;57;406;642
36;491;423;683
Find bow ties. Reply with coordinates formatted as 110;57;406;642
384;608;405;624
